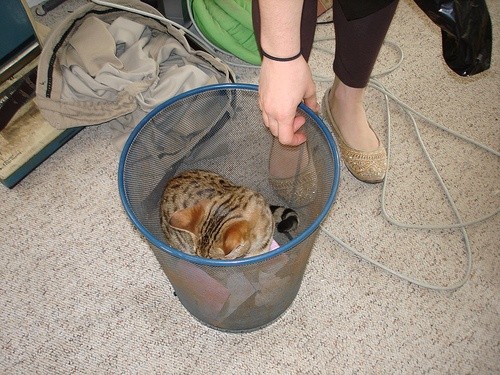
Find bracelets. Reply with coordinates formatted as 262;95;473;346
260;47;302;62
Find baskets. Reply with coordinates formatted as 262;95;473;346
119;83;341;333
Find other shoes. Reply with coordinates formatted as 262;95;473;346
322;88;387;184
268;133;318;208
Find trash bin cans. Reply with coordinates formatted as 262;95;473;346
118;84;340;332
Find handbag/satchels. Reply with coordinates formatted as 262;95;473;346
412;0;492;77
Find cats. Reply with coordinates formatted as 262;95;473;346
158;169;299;261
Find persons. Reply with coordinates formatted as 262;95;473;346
251;0;401;183
